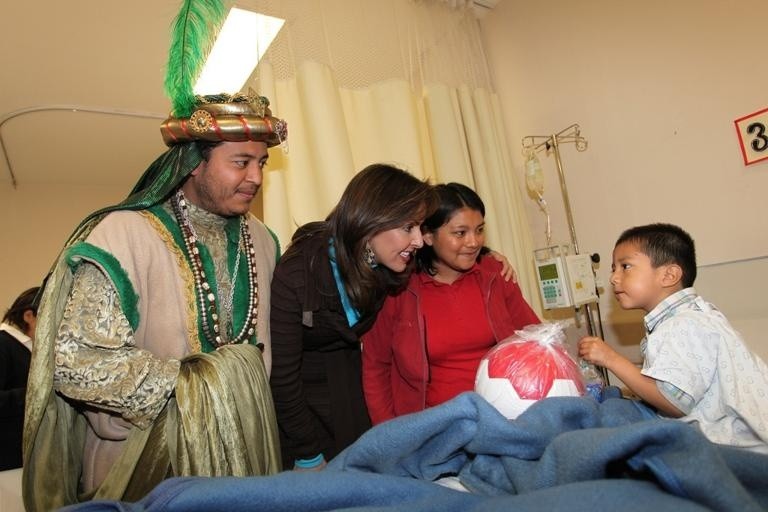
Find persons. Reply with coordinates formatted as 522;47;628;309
21;84;297;511
360;182;543;424
270;163;519;472
1;287;41;470
579;222;768;453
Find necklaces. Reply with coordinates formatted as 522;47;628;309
170;198;253;350
172;189;259;348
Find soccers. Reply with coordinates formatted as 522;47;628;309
475;336;586;420
